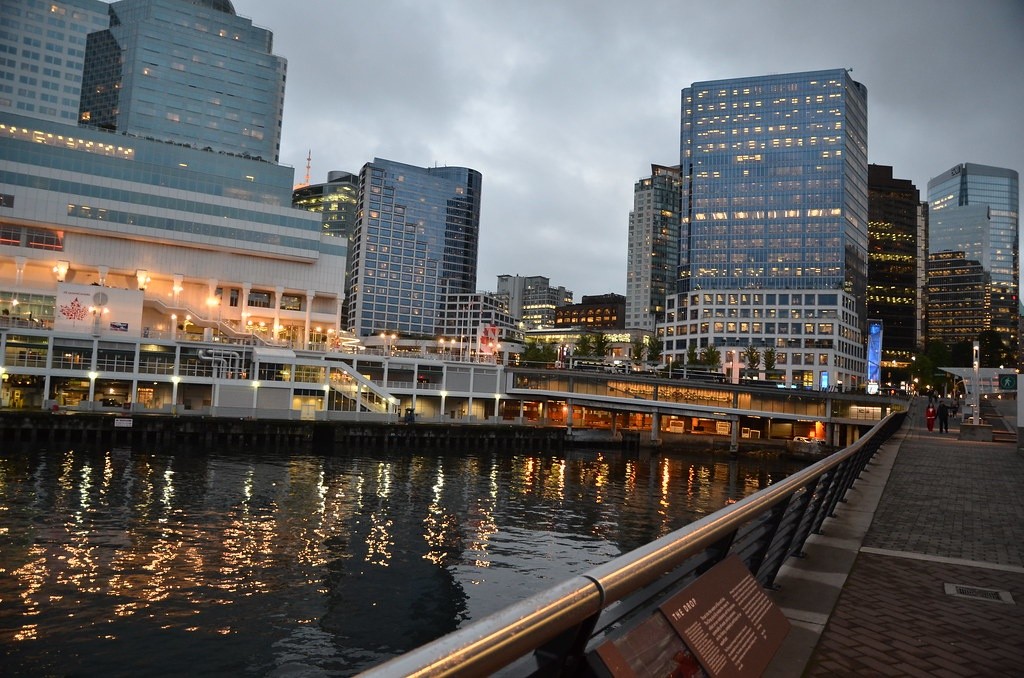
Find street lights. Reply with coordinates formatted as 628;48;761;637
379;333;387;356
388;335;397;357
726;349;736;385
462;335;470;362
312;326;321;349
207;297;221;337
970;339;981;425
86;306;109;370
440;338;446;360
449;339;456;352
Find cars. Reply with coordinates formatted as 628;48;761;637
100;398;117;407
19;350;41;361
17;378;33;388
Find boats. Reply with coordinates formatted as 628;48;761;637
785;439;822;462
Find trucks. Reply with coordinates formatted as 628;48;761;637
669;419;760;440
501;400;652;429
137;388;154;404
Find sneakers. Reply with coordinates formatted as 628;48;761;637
928;431;933;434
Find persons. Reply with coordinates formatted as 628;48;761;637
934;390;938;402
937;400;955;434
928;390;933;402
925;402;936;433
951;399;958;420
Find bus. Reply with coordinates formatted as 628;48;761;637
574;360;633;374
654;364;725;384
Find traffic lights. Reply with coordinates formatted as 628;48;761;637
561;343;568;369
